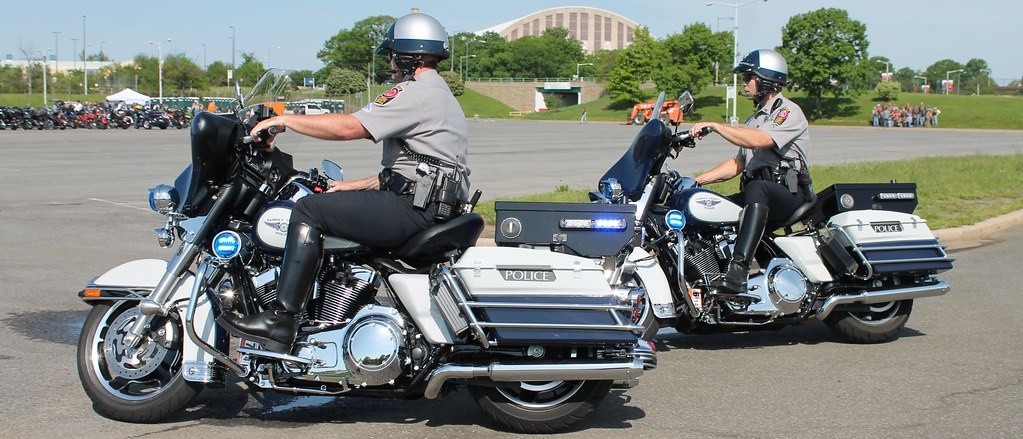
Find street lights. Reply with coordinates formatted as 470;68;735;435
371;46;376;85
980;69;991;86
459;55;477;80
466;40;486;81
714;17;735;86
946;69;964;95
82;16;88;94
52;31;61;74
228;26;235;68
149;40;172;105
201;44;207;70
268;47;281;67
876;60;888;82
706;1;771;128
577;63;594;81
38;48;52;104
914;76;927;93
71;39;79;69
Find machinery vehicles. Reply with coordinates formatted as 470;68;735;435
631;100;684;125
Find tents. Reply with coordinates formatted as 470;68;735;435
106;88;149;105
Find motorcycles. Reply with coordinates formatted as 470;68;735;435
77;67;658;433
588;89;956;344
0;103;194;130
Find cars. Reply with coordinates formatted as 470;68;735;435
382;80;397;86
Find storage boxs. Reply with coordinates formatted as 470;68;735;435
495;201;639;257
455;247;635;347
799;182;918;227
827;207;955;281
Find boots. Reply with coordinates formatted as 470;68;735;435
711;204;767;293
216;219;322;354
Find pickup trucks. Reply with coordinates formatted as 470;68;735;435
285;103;330;115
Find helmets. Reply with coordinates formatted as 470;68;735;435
731;49;788;85
374;12;450;60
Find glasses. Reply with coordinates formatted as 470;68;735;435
742;73;759;81
387;51;405;60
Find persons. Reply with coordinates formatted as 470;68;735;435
872;102;941;127
73;99;216;113
581;108;586;122
689;50;810;293
216;13;470;355
